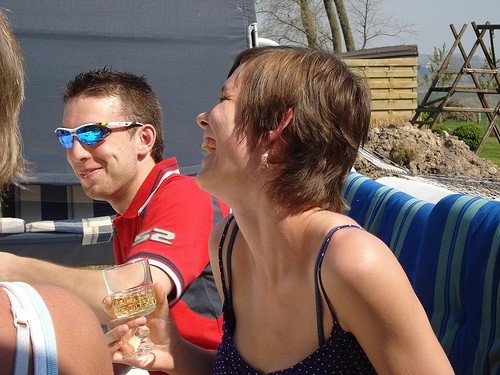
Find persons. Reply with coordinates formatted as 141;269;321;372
103;45;456;375
0;8;113;375
0;66;232;375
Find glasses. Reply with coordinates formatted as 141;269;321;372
54;122;160;149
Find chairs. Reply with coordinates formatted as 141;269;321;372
343;172;500;375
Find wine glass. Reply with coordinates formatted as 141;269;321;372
102;258;169;361
